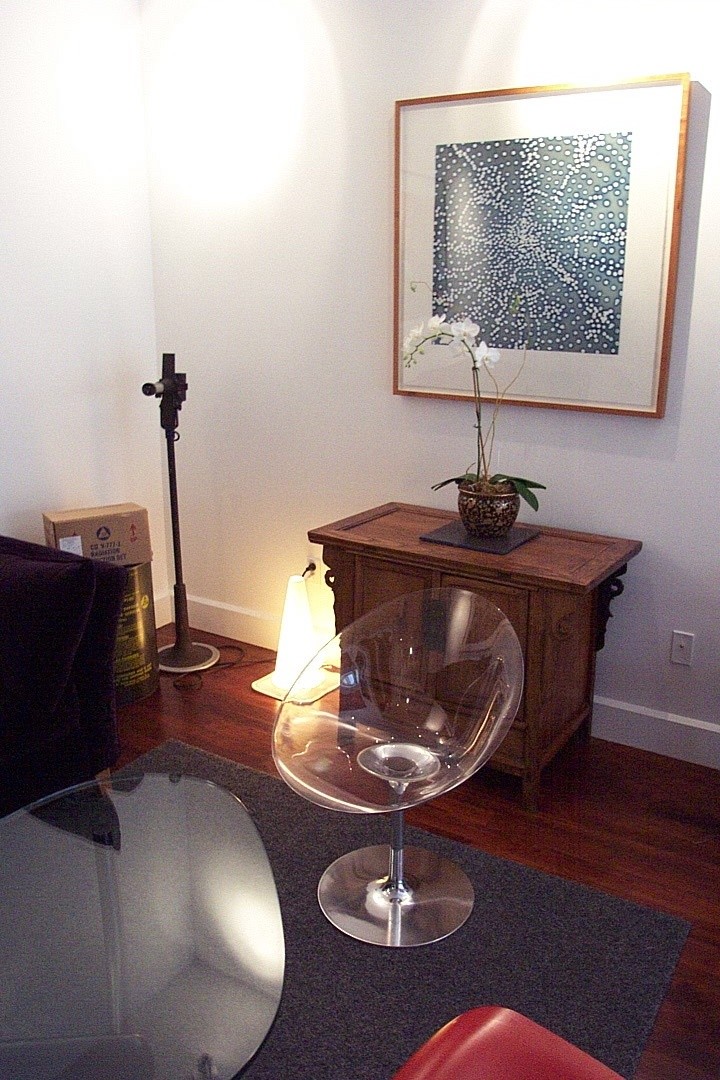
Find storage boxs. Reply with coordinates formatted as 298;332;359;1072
43;502;153;566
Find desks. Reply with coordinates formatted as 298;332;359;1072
0;773;286;1080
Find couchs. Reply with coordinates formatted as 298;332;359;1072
0;535;126;817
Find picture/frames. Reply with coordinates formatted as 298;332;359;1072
393;72;691;418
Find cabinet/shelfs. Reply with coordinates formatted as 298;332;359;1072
308;502;642;812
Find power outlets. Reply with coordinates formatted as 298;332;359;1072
308;556;322;579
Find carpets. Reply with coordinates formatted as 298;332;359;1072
114;738;689;1080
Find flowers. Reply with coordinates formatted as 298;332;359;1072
401;280;547;511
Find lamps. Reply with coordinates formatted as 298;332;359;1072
250;564;340;706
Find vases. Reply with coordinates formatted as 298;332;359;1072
458;486;520;537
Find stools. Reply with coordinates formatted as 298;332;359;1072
270;586;525;948
393;1007;624;1080
